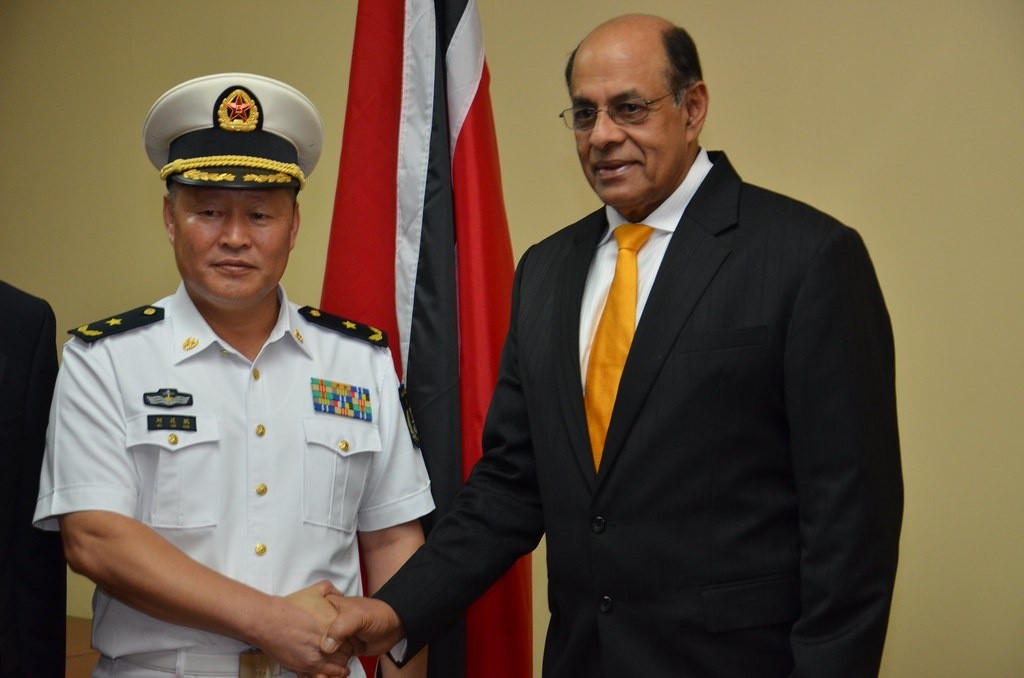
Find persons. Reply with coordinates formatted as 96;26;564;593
1;278;73;677
31;70;436;678
321;11;908;678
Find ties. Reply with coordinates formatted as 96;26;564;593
583;223;658;476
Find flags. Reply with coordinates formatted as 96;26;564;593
312;1;533;675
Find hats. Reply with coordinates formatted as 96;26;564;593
142;72;325;192
